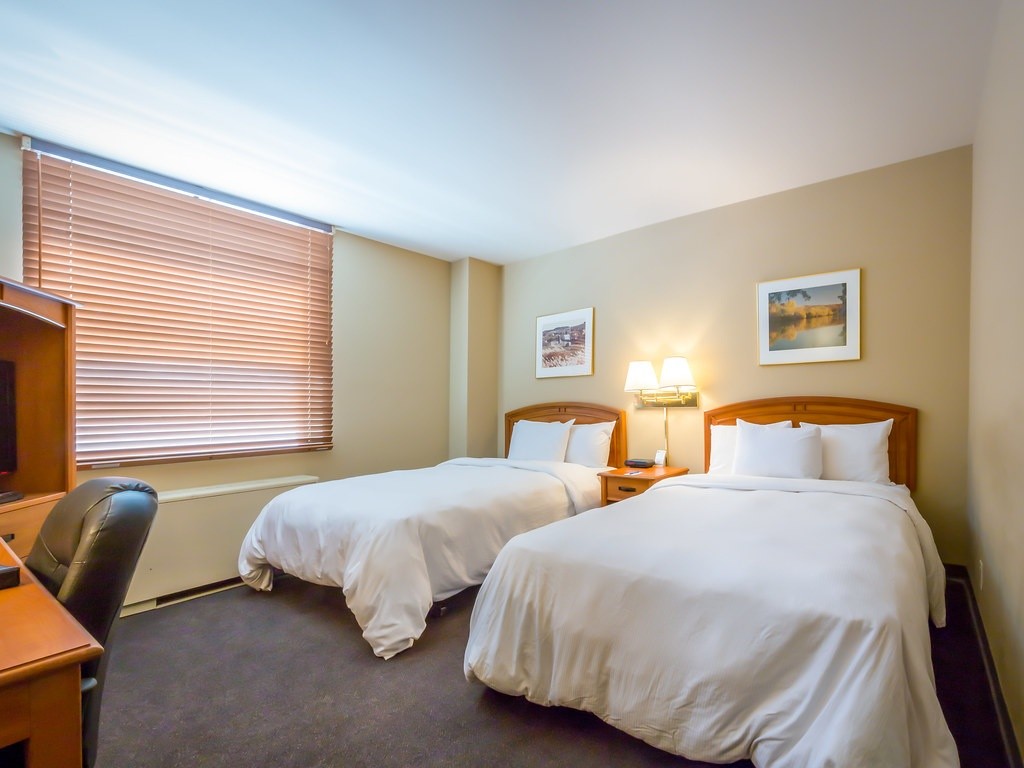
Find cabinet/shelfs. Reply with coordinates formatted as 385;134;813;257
0;277;85;563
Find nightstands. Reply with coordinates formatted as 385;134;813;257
596;465;690;508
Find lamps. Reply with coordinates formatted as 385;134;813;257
622;356;700;408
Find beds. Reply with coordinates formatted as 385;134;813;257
465;395;961;768
241;400;628;660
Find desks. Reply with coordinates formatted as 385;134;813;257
0;536;106;768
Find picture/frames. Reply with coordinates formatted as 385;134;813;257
534;307;595;378
755;267;863;366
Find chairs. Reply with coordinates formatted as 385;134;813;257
0;475;160;768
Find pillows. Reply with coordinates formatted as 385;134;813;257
559;420;617;468
798;418;895;482
733;417;824;480
707;420;792;474
509;418;576;464
